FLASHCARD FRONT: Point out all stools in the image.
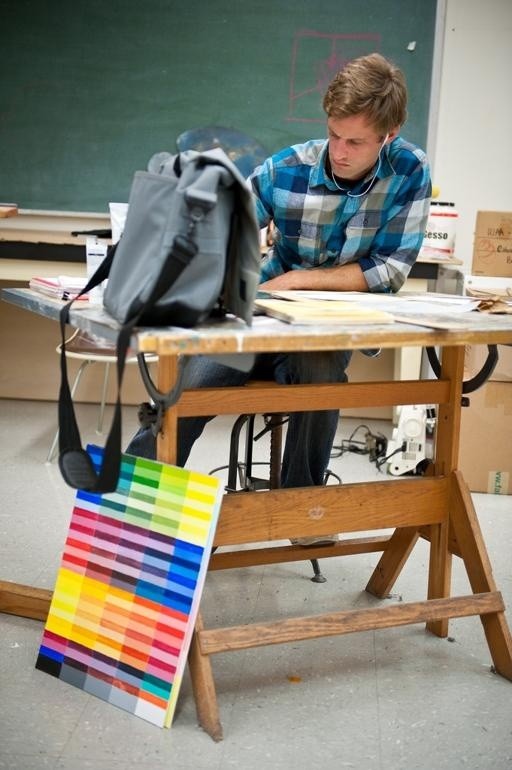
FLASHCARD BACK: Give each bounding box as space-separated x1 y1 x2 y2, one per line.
211 373 348 584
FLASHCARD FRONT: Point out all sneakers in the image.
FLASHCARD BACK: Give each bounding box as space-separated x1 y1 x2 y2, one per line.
289 532 338 547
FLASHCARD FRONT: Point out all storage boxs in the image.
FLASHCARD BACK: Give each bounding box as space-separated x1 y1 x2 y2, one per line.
471 209 511 277
434 344 512 495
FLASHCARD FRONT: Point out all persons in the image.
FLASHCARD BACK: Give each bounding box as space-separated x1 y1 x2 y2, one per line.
123 54 433 549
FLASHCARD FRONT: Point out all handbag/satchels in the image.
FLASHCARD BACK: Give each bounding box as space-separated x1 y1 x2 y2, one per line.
56 151 262 498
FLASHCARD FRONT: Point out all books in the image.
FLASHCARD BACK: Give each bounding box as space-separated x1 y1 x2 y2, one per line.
256 289 399 302
251 300 397 326
30 275 91 301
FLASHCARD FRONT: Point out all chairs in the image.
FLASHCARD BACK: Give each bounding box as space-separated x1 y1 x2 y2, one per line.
44 327 159 467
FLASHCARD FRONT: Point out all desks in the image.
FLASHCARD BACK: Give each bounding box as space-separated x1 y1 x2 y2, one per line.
0 287 512 743
